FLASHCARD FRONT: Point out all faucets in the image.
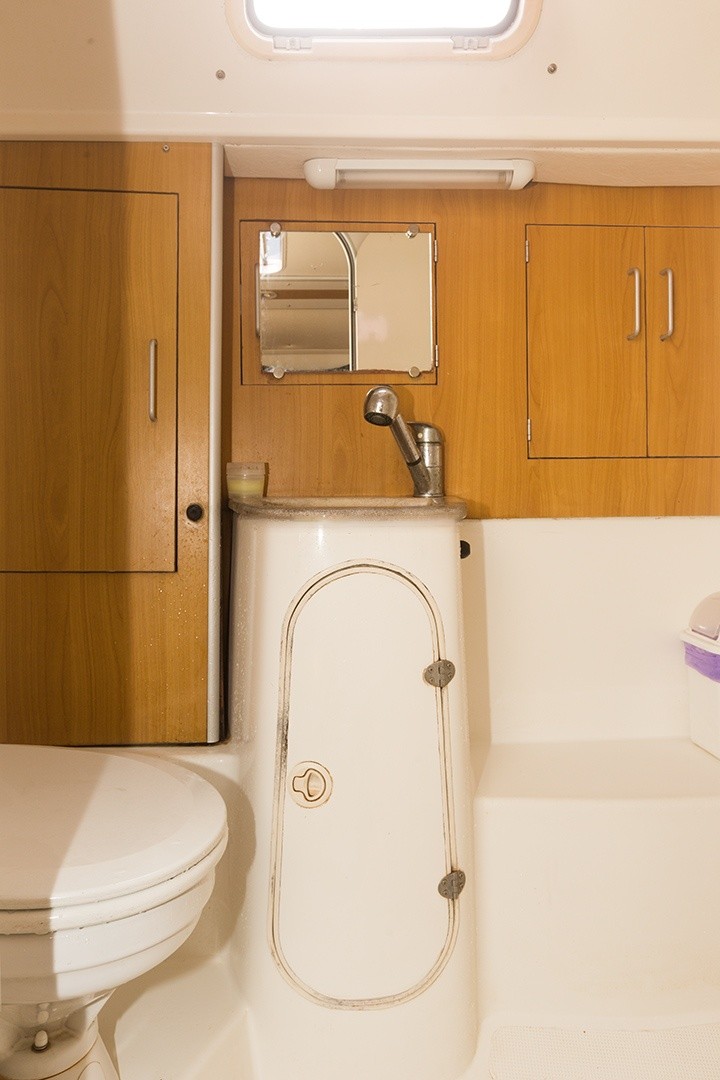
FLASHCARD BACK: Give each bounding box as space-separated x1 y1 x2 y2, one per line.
363 385 445 498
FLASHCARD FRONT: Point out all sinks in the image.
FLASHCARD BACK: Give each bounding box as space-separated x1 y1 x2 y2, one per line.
231 495 469 523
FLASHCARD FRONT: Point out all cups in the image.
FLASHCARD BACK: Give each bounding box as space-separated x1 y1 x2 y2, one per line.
225 461 265 500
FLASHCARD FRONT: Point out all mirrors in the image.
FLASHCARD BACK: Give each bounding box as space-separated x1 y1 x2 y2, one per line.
255 224 435 379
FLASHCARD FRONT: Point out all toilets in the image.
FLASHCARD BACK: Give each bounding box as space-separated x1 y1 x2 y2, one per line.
0 740 230 1080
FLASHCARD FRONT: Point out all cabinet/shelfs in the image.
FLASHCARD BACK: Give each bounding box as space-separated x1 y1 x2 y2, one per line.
0 138 226 746
223 177 720 517
